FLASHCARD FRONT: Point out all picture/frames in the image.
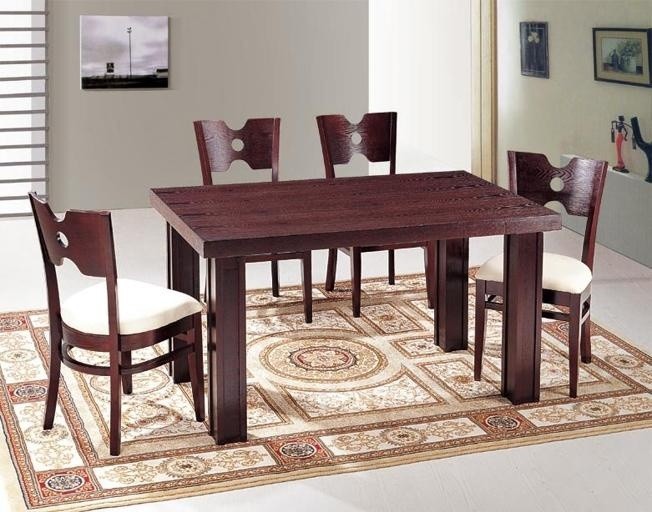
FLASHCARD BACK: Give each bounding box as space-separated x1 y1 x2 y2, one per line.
518 21 549 80
591 27 651 87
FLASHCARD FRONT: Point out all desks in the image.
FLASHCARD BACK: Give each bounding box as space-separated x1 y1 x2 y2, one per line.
148 169 564 446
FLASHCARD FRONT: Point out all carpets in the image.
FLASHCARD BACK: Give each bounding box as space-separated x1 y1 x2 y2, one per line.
0 266 652 510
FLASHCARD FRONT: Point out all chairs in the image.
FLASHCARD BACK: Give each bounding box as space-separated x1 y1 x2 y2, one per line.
193 118 312 322
316 113 436 317
27 191 204 456
470 149 608 400
